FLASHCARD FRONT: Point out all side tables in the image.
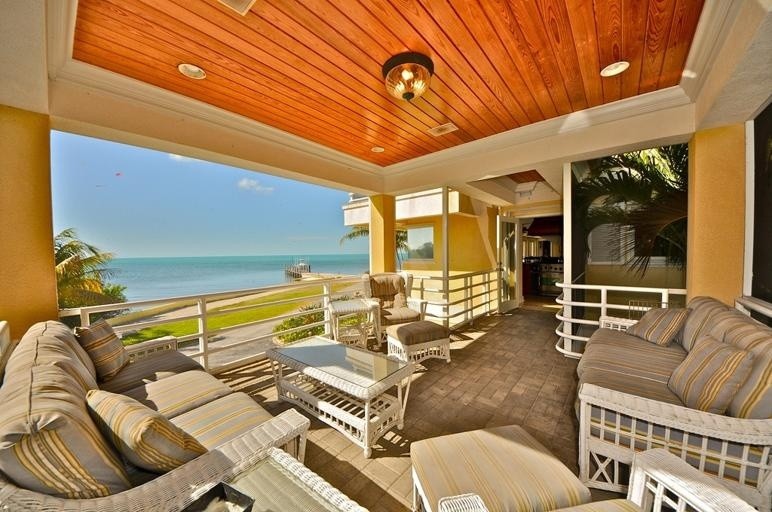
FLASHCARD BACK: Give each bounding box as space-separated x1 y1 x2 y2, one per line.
329 299 375 342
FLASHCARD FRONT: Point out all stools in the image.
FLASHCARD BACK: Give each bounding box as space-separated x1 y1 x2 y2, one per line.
387 320 452 366
412 426 592 510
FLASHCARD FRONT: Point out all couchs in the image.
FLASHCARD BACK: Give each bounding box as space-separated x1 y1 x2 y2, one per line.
574 296 770 494
0 319 310 512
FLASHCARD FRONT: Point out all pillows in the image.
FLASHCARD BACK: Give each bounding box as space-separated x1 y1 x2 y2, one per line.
85 389 209 475
625 308 691 348
667 335 754 415
74 317 131 382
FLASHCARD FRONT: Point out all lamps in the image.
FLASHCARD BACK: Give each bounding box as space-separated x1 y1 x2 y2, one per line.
383 53 434 103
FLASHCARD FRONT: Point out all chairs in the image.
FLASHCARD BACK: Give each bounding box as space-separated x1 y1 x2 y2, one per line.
363 272 428 348
